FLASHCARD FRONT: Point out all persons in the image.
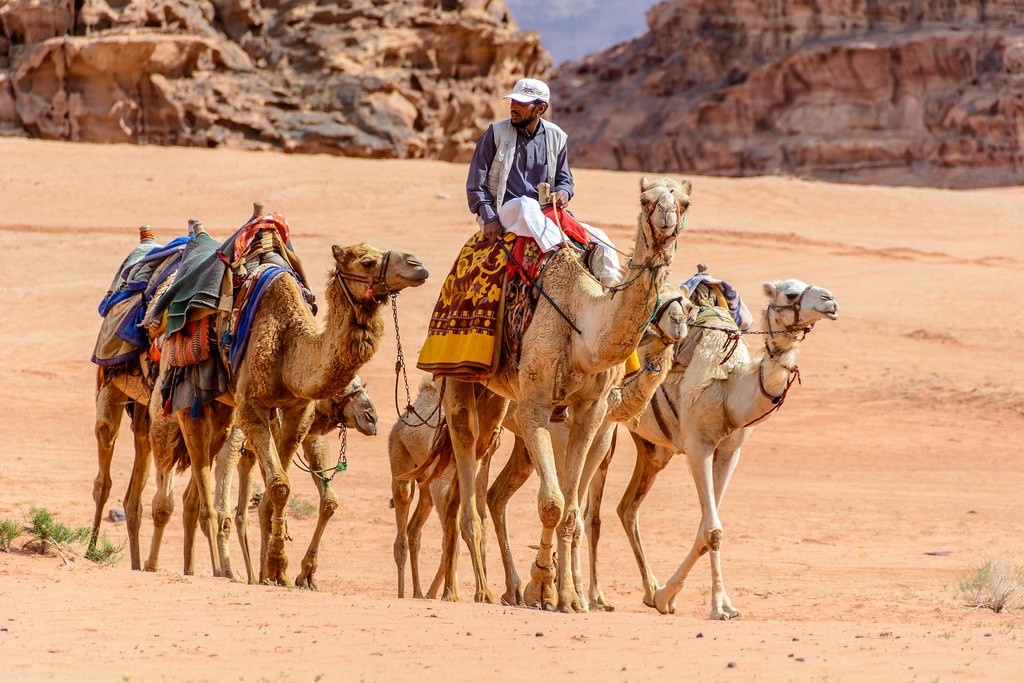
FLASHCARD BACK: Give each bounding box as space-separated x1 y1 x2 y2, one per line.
466 78 627 294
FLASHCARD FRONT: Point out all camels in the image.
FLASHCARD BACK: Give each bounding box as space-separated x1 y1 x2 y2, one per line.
81 202 429 594
388 176 840 620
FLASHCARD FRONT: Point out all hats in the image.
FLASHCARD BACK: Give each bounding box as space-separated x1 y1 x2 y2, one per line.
502 78 551 105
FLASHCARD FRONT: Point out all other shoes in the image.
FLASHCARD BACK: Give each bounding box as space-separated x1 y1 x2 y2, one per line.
583 241 604 280
